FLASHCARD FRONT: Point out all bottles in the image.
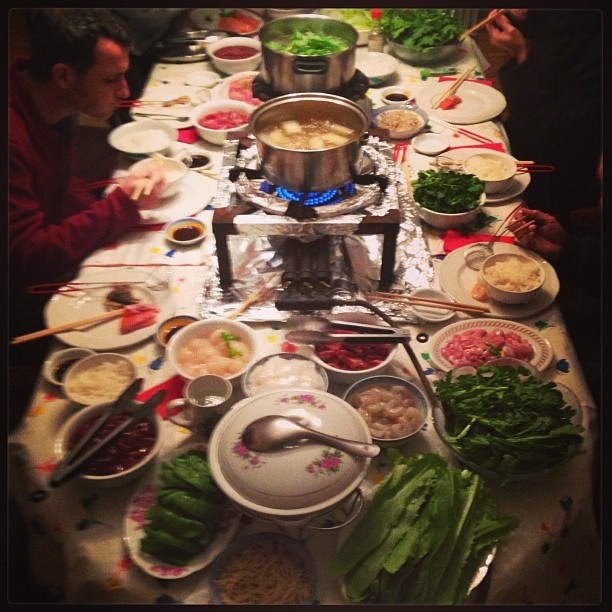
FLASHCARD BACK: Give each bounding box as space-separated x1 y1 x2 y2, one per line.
368 9 384 52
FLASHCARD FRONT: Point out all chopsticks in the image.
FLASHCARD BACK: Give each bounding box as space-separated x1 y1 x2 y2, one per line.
228 272 275 320
130 172 157 201
431 66 476 110
10 309 123 345
369 291 491 316
459 8 508 39
429 160 534 165
504 214 535 234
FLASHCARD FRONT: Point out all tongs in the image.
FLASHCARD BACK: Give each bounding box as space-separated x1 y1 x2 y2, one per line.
48 377 166 489
286 315 411 345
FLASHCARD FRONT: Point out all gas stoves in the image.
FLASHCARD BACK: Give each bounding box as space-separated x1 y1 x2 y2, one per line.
252 69 369 103
237 142 389 217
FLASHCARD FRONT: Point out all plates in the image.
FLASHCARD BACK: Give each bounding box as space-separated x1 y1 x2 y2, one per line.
42 347 96 385
220 9 265 35
371 104 429 140
180 151 213 171
380 86 414 104
43 277 163 351
211 71 264 110
330 480 497 605
267 8 300 18
156 17 208 63
184 69 220 88
103 167 215 224
208 532 318 605
439 242 561 318
129 85 211 129
428 318 553 382
107 120 179 156
156 315 199 349
411 132 449 155
415 80 507 124
436 148 531 203
164 217 208 246
280 14 329 19
409 288 457 322
123 443 244 580
432 358 583 482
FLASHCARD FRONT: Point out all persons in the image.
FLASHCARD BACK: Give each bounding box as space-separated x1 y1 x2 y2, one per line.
506 152 604 358
485 7 603 209
7 8 167 384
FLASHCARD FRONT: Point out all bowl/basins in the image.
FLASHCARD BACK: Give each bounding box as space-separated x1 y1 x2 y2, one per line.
309 312 398 385
242 352 329 399
413 191 486 228
465 153 517 195
481 252 546 305
207 37 262 76
127 157 187 199
343 374 428 448
207 388 372 530
355 28 377 46
54 401 166 489
191 99 253 146
386 38 459 65
62 353 137 407
357 51 399 87
165 317 259 386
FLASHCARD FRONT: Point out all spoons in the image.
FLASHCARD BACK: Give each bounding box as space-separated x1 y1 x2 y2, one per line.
129 112 188 122
242 415 381 458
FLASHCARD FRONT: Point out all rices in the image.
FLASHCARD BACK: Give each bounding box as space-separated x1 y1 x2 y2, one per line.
484 257 541 292
67 362 132 402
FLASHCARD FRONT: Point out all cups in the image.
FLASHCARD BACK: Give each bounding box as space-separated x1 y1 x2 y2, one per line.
165 375 233 432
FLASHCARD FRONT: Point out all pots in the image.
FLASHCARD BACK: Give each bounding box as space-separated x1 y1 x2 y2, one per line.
248 92 370 191
259 14 359 95
